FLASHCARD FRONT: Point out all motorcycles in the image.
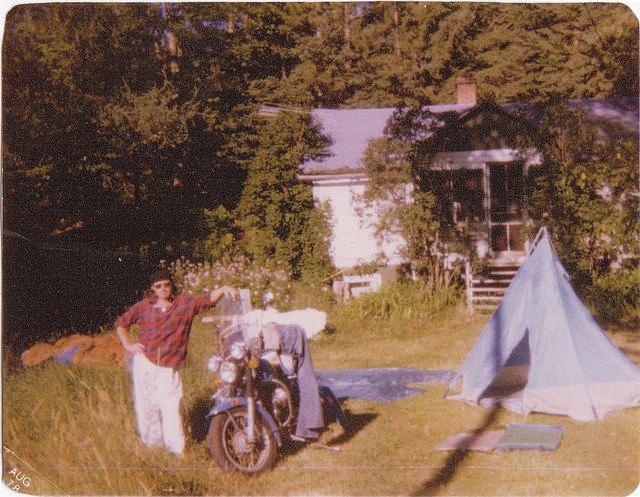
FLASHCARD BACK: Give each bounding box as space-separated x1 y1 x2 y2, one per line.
201 288 354 477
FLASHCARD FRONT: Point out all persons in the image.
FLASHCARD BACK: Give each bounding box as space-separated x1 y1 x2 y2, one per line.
112 265 240 465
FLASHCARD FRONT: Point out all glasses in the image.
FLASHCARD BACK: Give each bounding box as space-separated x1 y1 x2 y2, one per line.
152 283 171 289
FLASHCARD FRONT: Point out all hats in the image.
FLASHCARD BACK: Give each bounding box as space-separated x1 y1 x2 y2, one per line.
152 270 171 282
